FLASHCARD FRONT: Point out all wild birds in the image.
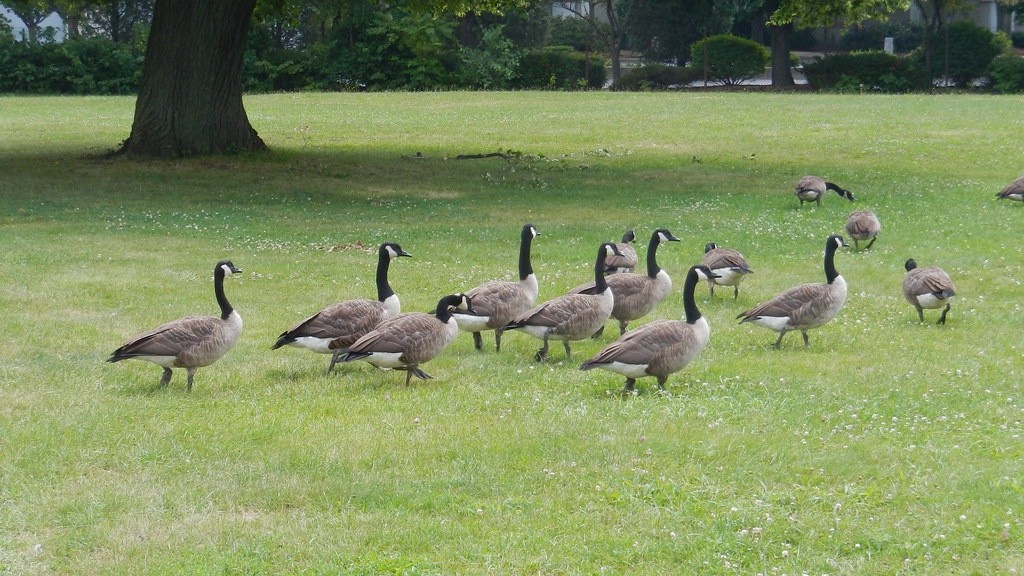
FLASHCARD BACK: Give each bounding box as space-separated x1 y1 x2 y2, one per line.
845 208 881 251
105 260 244 391
426 223 541 352
736 233 851 349
601 230 638 277
578 265 722 394
903 258 956 325
699 243 753 302
994 175 1024 204
334 293 478 388
496 242 625 363
792 175 857 208
565 229 681 339
269 242 412 373
